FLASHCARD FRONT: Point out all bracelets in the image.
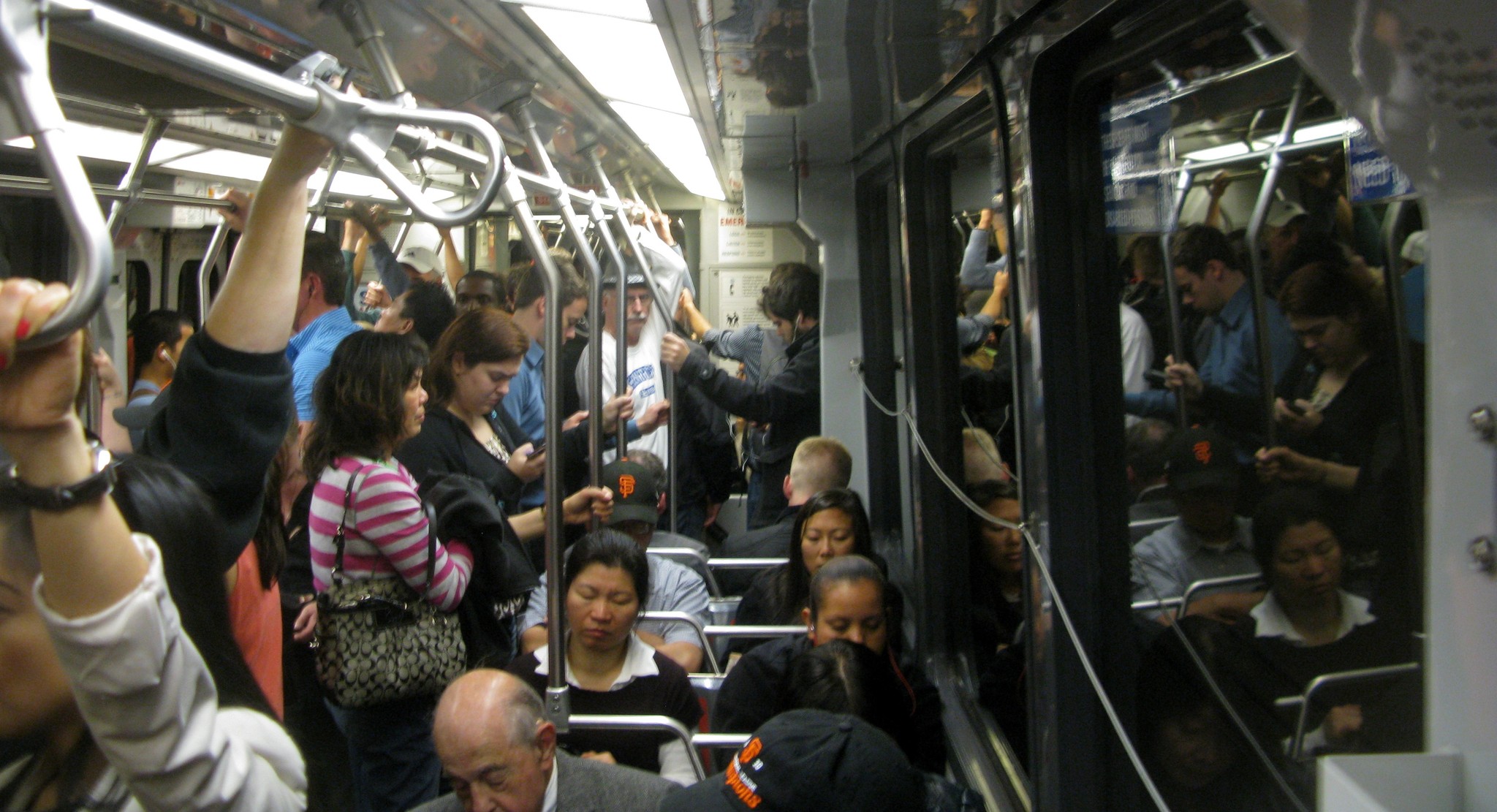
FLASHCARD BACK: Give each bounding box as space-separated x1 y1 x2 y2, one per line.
0 436 117 512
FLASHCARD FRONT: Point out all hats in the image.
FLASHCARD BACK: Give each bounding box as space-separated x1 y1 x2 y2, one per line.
601 257 646 284
1170 425 1241 491
599 457 659 523
660 709 915 812
624 451 668 490
394 247 443 276
1401 230 1426 264
1264 200 1308 226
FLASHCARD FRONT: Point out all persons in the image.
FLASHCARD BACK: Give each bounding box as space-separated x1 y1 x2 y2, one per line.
711 555 946 773
720 437 852 595
408 668 682 812
660 709 925 812
518 460 710 673
128 309 195 452
956 188 1151 753
504 529 704 773
660 261 820 537
719 489 904 672
211 189 693 812
0 277 310 812
74 73 365 716
1118 151 1429 812
784 639 985 812
628 449 710 588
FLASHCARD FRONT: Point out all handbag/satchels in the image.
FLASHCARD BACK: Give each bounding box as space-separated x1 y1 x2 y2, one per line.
308 467 465 711
